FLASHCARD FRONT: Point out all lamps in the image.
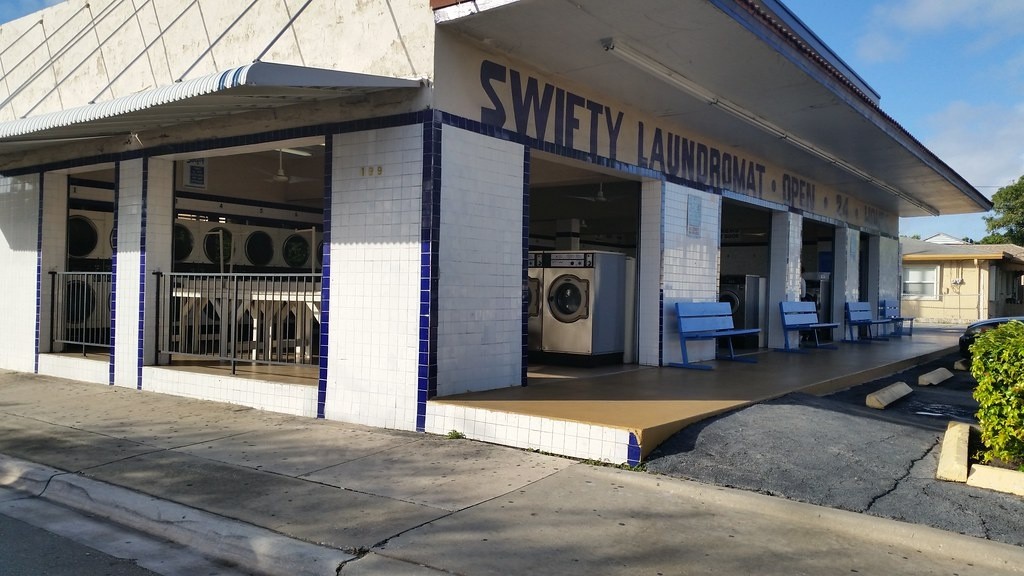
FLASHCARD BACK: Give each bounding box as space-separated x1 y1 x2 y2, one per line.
599 38 940 218
276 149 314 157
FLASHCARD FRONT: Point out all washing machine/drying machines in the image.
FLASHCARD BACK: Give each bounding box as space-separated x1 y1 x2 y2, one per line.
65 179 325 342
718 275 758 350
528 250 626 356
801 271 831 330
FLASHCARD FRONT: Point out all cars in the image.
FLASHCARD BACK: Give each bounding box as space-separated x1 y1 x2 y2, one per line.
958 315 1024 362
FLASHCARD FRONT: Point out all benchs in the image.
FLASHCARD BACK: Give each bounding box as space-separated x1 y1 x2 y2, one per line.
668 302 762 369
841 301 892 343
880 299 915 337
774 302 840 352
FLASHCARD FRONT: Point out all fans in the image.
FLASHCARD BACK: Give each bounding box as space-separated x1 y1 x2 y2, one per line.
250 148 324 183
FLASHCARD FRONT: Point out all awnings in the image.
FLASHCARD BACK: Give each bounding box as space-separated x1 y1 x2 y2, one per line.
0 62 426 154
